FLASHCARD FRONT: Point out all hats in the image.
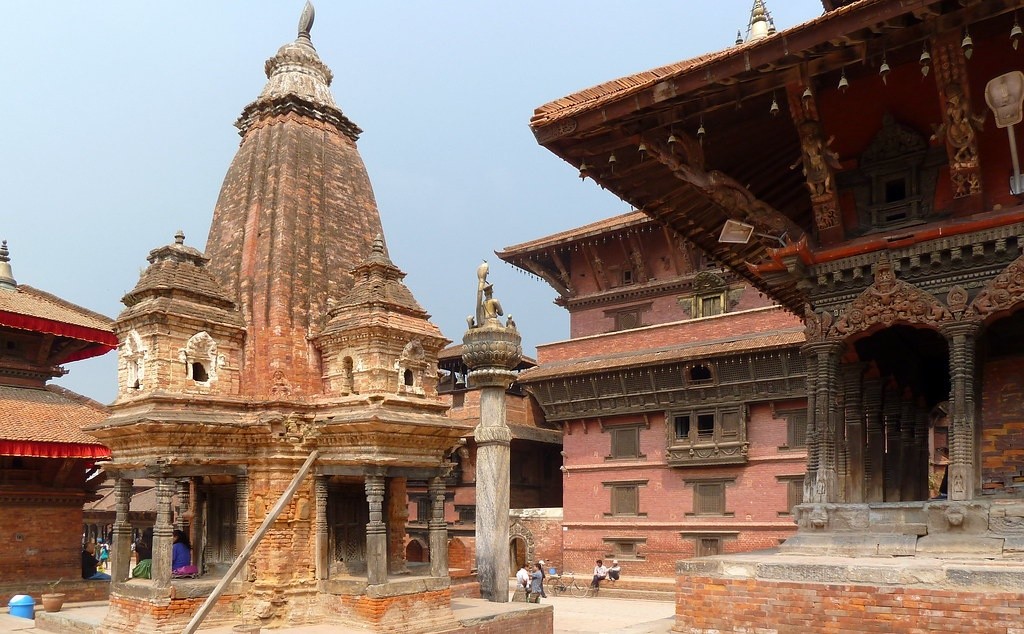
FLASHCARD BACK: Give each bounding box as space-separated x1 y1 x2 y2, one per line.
102 545 106 547
613 560 618 564
539 561 545 565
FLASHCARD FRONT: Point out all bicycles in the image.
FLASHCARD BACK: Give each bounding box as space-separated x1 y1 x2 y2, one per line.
547 571 588 598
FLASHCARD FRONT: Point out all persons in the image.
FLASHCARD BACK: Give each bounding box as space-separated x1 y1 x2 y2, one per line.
100 545 109 569
591 560 607 588
82 543 111 580
481 283 503 327
528 563 543 601
538 560 547 598
516 563 530 589
606 560 621 581
172 530 190 570
131 543 152 578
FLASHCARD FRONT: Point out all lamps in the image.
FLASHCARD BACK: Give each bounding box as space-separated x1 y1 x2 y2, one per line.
984 72 1024 193
718 220 779 246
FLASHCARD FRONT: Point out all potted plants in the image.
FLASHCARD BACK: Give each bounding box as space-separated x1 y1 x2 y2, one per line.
41 577 66 612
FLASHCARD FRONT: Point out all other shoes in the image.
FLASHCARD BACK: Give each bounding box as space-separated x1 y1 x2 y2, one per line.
612 578 615 582
542 595 547 598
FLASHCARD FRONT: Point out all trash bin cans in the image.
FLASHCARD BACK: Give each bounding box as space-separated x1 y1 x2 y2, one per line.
8 594 36 619
